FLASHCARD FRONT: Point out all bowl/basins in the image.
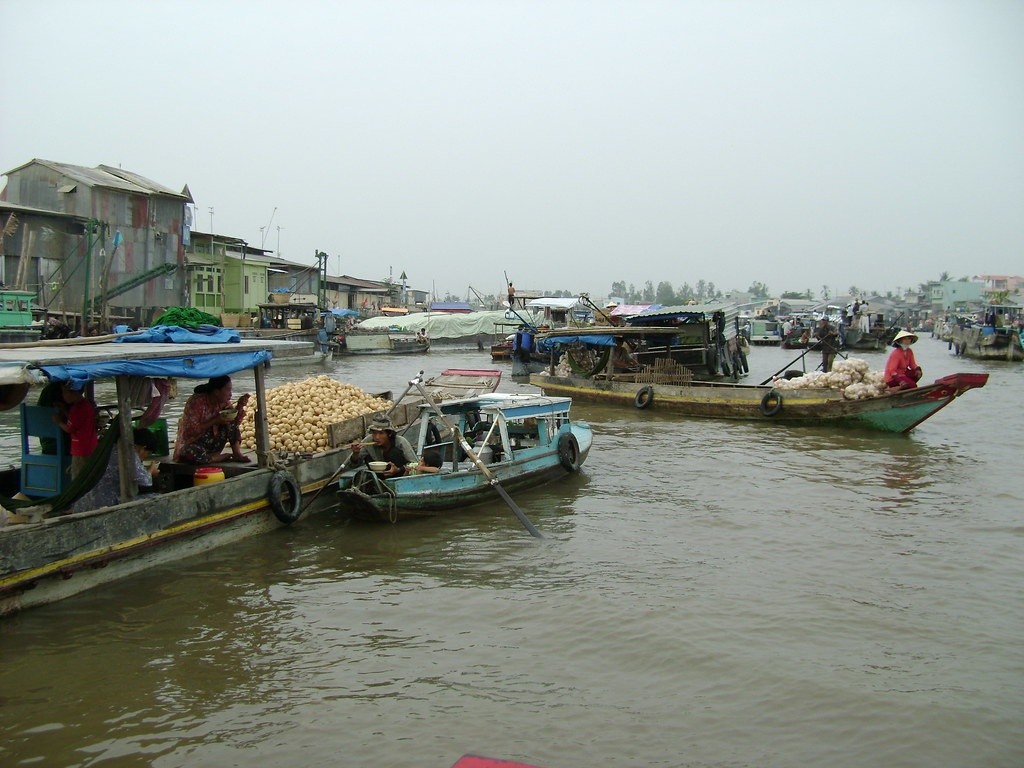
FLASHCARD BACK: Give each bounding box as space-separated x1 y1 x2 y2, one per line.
219 409 238 421
368 461 389 470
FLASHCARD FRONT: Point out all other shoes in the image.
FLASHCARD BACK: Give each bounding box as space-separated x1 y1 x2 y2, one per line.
913 367 921 378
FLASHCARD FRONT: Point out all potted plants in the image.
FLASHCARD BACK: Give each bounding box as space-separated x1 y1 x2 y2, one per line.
238 312 252 327
221 311 239 328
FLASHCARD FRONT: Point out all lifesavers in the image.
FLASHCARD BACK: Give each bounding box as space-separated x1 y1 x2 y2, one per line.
733 363 739 379
469 411 482 431
633 385 654 410
722 361 731 376
706 347 718 375
268 470 301 523
740 352 750 373
761 392 782 416
558 432 580 473
733 351 743 374
713 310 726 335
427 422 442 445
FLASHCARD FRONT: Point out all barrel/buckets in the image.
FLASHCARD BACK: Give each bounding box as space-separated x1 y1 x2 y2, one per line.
134 418 169 457
194 467 225 486
472 441 493 464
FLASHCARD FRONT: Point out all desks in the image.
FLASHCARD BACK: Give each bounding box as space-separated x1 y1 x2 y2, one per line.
256 302 314 330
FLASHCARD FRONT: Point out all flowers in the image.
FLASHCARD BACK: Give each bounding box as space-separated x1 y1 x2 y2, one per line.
272 288 291 294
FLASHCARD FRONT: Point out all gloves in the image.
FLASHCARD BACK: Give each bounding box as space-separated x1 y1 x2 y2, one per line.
900 381 910 390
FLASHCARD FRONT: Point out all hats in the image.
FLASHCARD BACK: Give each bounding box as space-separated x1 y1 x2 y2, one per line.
891 330 918 347
606 302 617 308
367 413 405 433
821 316 829 321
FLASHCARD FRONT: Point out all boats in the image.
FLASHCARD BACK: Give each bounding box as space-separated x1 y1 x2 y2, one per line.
0 342 502 617
491 296 1024 379
240 303 351 349
529 327 989 434
324 399 594 523
0 290 146 347
324 328 430 353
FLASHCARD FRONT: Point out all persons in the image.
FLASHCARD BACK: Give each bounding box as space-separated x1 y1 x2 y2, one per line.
813 316 840 372
362 297 429 317
735 297 880 351
174 376 251 466
260 307 361 358
887 303 1024 339
508 282 516 309
416 328 429 344
348 413 443 481
885 330 923 390
36 382 98 515
71 428 160 513
475 431 498 464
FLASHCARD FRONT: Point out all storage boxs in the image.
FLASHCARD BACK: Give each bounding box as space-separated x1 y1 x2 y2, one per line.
284 318 301 330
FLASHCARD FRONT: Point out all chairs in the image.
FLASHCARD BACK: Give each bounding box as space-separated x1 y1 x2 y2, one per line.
18 402 72 499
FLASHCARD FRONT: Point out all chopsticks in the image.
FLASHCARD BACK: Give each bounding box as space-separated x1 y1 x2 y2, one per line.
224 399 240 408
355 442 377 445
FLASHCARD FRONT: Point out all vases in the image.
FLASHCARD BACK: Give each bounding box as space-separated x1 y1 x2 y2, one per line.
272 293 290 304
